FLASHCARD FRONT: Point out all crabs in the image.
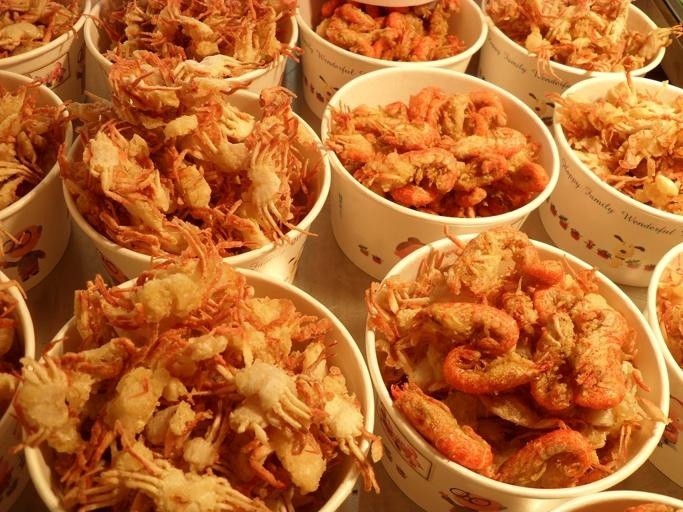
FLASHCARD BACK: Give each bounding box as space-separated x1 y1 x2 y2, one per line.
0 0 383 512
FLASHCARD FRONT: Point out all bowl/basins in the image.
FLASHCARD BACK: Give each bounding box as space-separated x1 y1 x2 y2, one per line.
552 490 682 511
297 2 491 120
59 84 332 288
363 230 670 512
316 63 564 282
471 1 668 136
1 266 38 510
1 70 74 294
2 1 83 102
642 243 683 489
20 265 378 510
537 74 683 285
84 0 299 108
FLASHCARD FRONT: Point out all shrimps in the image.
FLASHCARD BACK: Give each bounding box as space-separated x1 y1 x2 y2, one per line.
308 0 670 512
